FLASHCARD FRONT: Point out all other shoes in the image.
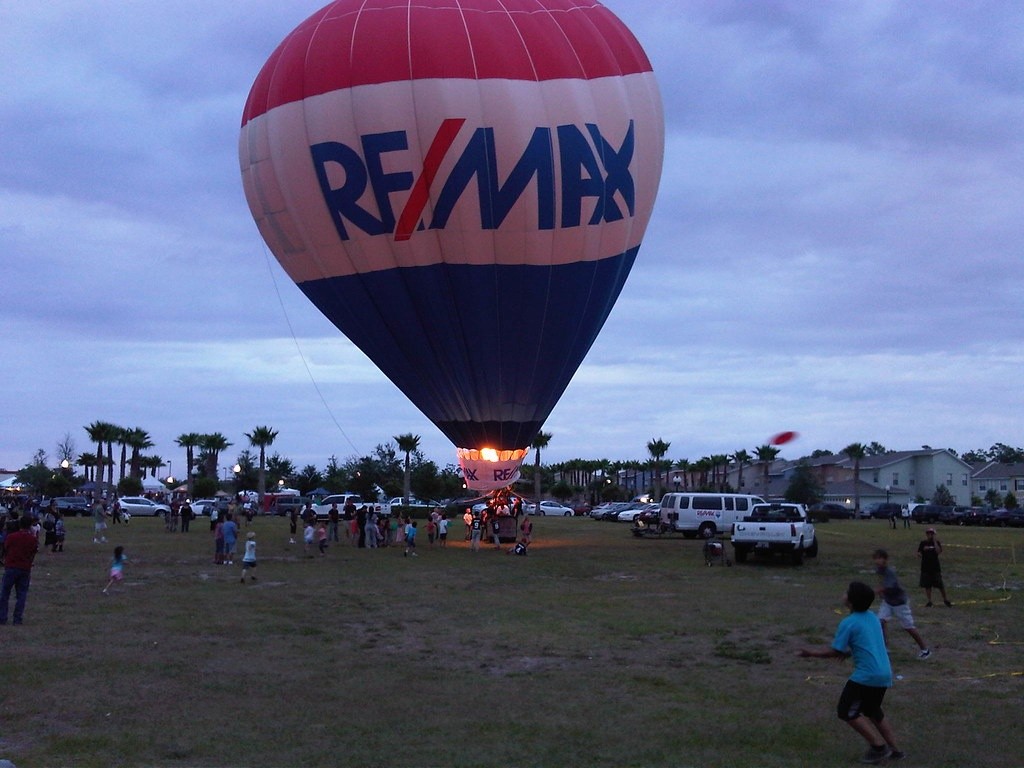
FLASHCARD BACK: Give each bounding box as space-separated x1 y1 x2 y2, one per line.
94 539 100 544
404 551 408 557
213 559 233 565
101 539 108 542
925 602 934 608
241 576 245 584
103 589 109 596
0 622 8 625
944 600 953 608
251 576 257 580
412 553 418 557
13 621 22 626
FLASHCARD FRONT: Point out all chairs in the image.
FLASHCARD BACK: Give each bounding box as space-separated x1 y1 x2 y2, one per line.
758 508 768 515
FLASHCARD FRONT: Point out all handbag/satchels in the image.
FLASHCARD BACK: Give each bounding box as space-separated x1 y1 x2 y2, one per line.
43 520 54 530
211 510 218 521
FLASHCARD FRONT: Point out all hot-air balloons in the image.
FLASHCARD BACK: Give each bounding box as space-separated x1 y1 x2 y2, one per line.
236 0 664 542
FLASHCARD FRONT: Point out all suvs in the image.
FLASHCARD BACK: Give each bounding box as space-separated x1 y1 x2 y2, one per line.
40 496 92 517
107 496 172 518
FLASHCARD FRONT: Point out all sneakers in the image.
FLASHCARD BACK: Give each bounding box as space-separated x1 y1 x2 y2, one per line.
860 747 892 763
917 648 932 660
890 750 906 761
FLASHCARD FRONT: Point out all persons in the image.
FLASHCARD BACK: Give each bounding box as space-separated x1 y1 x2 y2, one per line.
208 496 241 565
145 489 164 504
301 502 329 555
169 498 194 532
422 508 449 550
793 581 908 763
0 493 67 568
0 515 40 625
396 506 418 557
102 546 131 595
92 497 131 544
240 532 258 584
891 514 898 529
343 497 394 549
288 508 298 544
916 527 953 607
327 502 340 543
868 549 932 659
463 496 533 556
901 505 912 529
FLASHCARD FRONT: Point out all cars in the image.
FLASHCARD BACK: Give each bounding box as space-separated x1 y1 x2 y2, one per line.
178 499 218 520
391 494 659 527
810 503 1024 528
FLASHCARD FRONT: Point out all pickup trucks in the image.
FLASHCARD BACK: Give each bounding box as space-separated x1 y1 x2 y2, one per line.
300 495 391 519
731 503 818 567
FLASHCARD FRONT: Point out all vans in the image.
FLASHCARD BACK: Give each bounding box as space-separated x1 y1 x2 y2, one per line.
269 496 315 518
659 492 768 540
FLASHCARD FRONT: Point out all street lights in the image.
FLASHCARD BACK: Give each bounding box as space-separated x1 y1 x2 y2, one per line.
884 485 891 502
222 466 227 483
167 460 172 478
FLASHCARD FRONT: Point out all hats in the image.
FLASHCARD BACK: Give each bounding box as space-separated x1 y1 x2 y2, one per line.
926 528 936 534
522 538 526 543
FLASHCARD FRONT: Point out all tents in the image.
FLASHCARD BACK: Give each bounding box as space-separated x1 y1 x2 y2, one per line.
142 475 166 498
